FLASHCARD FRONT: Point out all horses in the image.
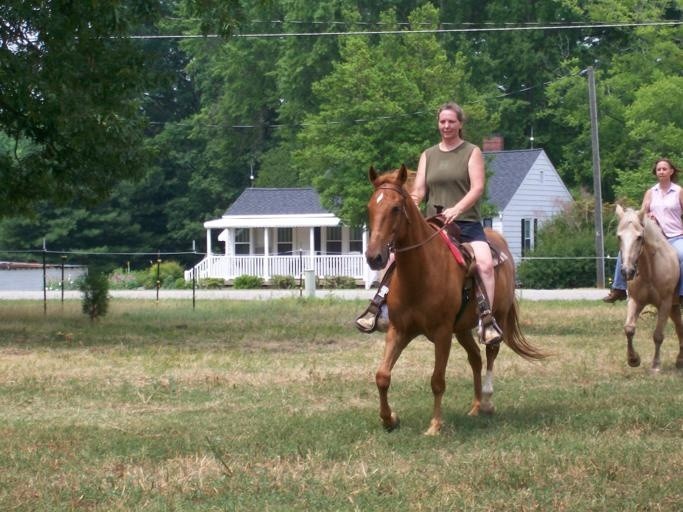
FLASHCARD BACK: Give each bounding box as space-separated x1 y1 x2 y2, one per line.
365 162 550 436
615 203 683 375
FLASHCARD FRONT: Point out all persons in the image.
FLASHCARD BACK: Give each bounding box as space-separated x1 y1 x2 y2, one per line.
603 157 682 305
355 102 504 344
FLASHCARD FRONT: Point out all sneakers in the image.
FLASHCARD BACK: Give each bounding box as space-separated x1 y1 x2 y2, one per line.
478 318 504 346
603 288 626 304
356 311 388 333
